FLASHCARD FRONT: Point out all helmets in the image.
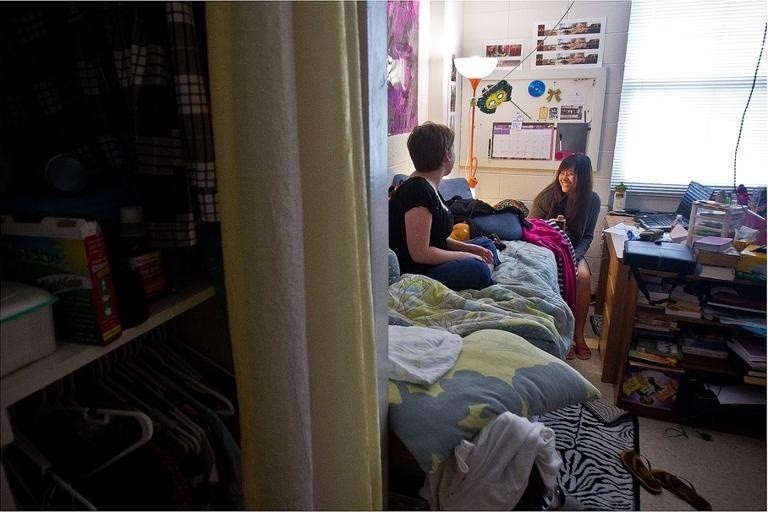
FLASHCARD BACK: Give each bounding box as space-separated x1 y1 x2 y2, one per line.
493 199 529 218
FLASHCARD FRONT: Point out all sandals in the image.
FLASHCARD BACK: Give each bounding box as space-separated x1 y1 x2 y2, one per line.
567 336 591 360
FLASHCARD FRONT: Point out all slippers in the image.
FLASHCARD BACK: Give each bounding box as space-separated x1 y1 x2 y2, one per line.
653 469 711 510
621 449 661 494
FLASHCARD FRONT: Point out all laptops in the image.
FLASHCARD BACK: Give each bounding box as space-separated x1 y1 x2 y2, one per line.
636 180 715 231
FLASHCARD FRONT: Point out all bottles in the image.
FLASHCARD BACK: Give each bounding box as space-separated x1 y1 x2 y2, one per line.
671 215 685 233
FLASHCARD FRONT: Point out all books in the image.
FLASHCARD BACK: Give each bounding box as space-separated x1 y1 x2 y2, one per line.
628 236 767 386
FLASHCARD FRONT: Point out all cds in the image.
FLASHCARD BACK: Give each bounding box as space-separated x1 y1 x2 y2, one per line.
528 80 545 97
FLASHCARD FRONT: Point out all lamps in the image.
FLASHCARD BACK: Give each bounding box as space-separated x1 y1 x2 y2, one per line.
452 55 499 186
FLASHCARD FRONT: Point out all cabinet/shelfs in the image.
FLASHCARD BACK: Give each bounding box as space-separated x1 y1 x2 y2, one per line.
588 214 768 419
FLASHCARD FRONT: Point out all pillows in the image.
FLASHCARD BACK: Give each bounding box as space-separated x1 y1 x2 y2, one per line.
396 174 523 241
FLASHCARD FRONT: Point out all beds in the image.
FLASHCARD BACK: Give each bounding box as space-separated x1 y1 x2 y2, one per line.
383 214 569 511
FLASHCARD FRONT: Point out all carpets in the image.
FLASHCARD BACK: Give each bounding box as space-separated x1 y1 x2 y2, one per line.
525 400 641 511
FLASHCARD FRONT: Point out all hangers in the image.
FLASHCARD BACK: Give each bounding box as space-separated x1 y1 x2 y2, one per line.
9 305 240 511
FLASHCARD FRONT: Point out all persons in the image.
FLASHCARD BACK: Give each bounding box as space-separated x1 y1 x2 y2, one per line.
389 121 499 291
526 153 600 359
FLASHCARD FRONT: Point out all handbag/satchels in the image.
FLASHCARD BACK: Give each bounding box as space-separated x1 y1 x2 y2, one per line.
623 240 695 274
